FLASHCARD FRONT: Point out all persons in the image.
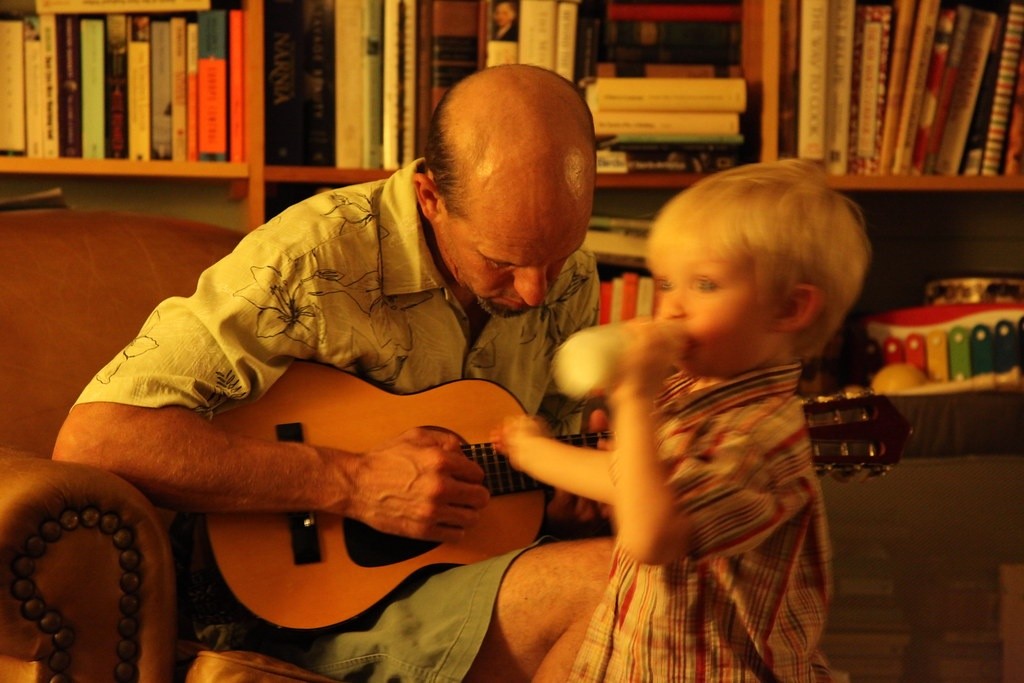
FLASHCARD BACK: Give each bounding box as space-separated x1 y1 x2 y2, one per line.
492 160 872 683
51 64 612 683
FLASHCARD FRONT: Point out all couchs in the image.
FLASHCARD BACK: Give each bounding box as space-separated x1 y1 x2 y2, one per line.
0 210 329 683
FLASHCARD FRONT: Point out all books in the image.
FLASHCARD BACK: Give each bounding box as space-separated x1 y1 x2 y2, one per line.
0 0 1024 323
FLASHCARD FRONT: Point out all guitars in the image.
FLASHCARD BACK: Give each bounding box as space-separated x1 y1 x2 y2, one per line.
203 356 915 637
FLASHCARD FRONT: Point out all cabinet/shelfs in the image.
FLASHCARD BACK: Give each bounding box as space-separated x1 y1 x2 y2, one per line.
0 0 1024 234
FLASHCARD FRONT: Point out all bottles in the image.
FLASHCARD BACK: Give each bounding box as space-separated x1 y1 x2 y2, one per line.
552 317 688 401
